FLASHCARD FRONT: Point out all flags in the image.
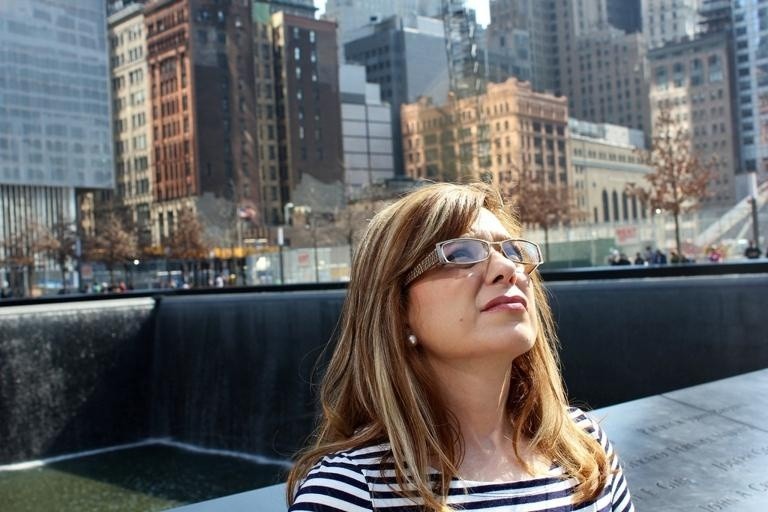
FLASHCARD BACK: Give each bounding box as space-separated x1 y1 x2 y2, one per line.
237 204 256 219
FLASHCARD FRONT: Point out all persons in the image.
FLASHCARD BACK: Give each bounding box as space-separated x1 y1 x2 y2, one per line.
58 284 71 294
285 180 637 512
608 246 696 266
0 280 20 298
745 239 762 259
81 271 228 293
708 246 722 262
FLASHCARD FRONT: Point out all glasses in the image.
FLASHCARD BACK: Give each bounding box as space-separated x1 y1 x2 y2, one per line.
402 238 544 286
